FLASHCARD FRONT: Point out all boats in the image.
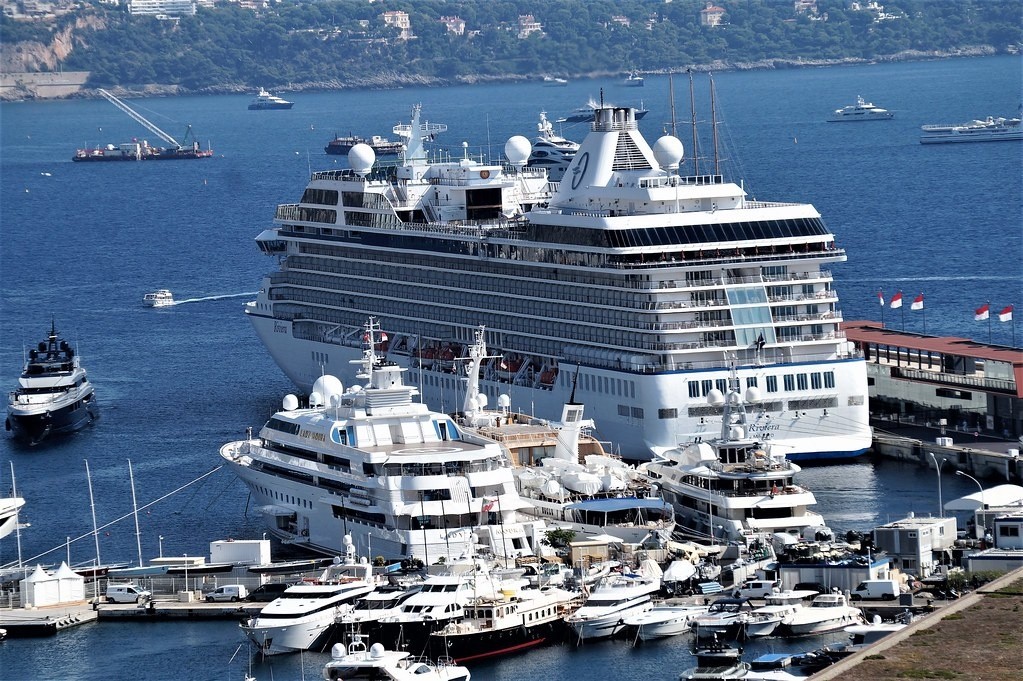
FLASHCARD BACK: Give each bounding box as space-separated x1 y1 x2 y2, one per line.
919 104 1023 144
543 76 567 88
142 289 174 308
324 131 402 156
540 366 560 387
216 314 547 572
6 311 99 445
566 99 650 123
494 359 520 380
0 351 1021 680
72 87 213 161
434 347 455 371
414 348 432 368
625 65 644 86
247 87 295 110
825 95 893 122
363 332 388 351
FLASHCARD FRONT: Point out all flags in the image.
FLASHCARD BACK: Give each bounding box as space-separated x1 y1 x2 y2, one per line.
482 499 494 514
451 362 456 373
382 333 388 342
501 359 507 370
878 291 884 306
889 291 902 309
755 334 765 352
363 332 369 342
998 305 1013 323
975 304 989 321
910 294 924 310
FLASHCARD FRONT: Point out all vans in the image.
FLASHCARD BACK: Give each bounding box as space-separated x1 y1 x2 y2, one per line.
732 580 781 599
794 581 833 601
850 580 901 601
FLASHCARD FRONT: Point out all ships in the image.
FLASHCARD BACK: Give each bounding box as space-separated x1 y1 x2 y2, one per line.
243 98 874 466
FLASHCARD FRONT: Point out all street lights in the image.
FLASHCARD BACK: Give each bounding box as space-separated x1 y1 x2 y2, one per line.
708 456 721 546
956 470 985 533
929 452 946 518
